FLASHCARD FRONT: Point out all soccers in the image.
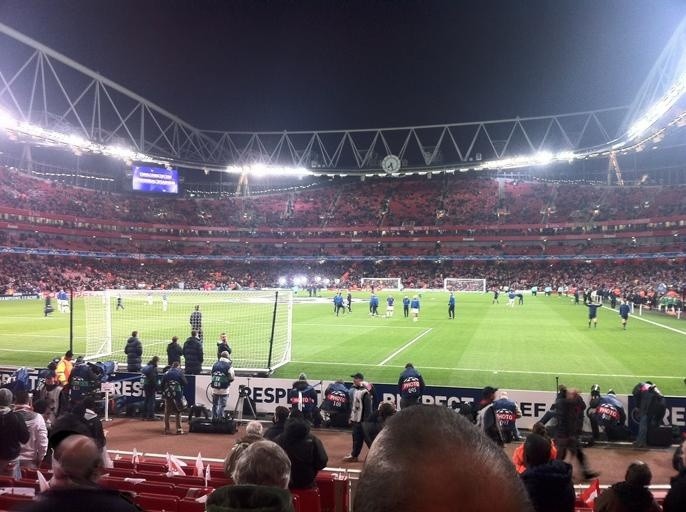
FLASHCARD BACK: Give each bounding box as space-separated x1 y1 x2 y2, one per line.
570 299 574 302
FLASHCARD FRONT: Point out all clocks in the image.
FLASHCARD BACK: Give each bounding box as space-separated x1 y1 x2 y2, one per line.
380 154 401 174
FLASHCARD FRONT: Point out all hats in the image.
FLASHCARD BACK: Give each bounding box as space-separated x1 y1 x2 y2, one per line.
500 391 508 400
482 386 498 398
351 373 364 380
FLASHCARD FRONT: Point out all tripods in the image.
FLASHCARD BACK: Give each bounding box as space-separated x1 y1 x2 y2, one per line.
234 398 257 418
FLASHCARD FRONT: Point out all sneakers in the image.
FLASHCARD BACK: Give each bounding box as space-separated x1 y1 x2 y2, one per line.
342 454 358 462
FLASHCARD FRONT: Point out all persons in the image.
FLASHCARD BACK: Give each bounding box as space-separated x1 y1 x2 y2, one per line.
511 372 686 512
358 172 686 331
0 330 358 512
1 159 357 319
190 305 203 343
357 363 511 462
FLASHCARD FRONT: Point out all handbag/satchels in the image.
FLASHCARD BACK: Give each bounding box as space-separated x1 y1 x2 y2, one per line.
188 417 237 435
173 395 188 412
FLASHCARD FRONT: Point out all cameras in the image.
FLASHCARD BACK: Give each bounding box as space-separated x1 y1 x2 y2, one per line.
238 385 247 398
590 384 601 397
